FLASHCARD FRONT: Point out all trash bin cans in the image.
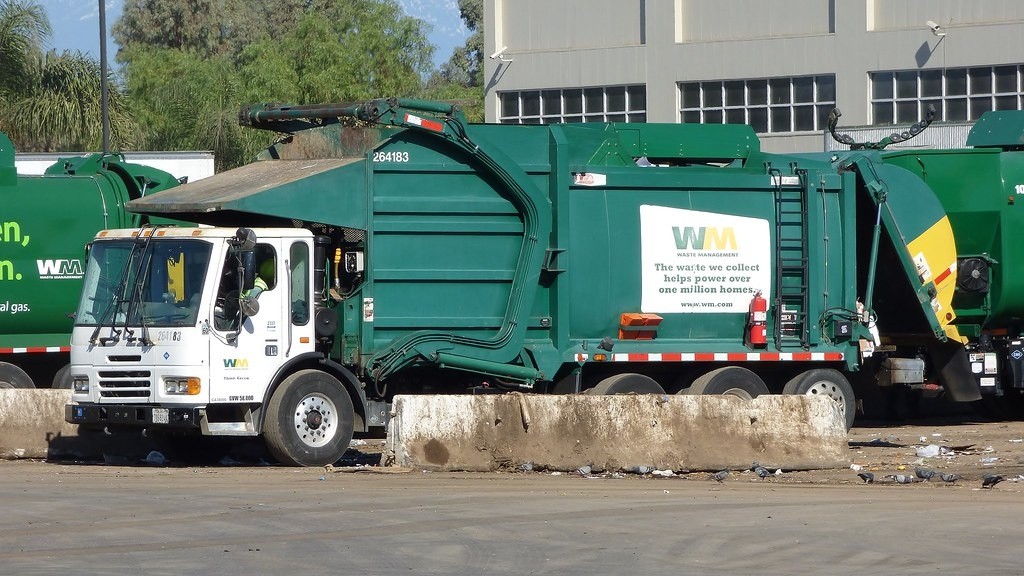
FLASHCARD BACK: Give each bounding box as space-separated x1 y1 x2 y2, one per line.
989 334 1024 390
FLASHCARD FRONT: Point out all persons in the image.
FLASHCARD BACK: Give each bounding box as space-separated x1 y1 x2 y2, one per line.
241 262 268 299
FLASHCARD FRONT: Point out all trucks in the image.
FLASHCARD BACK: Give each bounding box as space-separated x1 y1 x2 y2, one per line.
0 132 200 389
63 96 981 467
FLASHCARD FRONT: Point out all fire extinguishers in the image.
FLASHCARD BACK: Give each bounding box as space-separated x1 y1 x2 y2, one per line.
742 292 766 346
